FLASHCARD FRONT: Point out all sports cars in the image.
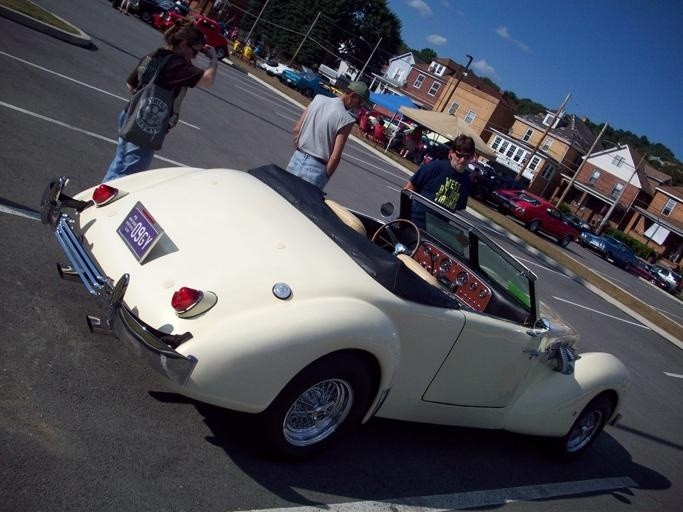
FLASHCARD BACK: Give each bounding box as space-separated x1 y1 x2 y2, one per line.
35 164 635 462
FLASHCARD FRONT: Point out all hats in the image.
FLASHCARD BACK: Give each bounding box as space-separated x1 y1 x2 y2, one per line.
347 81 373 109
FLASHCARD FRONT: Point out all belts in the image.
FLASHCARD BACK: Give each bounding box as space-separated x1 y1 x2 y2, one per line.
295 146 328 166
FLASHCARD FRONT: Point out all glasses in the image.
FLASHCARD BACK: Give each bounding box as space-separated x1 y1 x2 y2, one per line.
452 148 473 160
187 44 199 57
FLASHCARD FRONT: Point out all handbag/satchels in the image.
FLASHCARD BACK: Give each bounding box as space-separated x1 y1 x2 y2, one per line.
119 83 174 150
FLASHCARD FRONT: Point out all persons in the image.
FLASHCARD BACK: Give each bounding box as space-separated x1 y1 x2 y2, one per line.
98 19 217 186
119 0 132 17
355 110 369 138
405 134 475 248
249 45 256 66
111 1 121 11
374 120 387 146
284 81 374 192
399 125 422 159
390 127 405 148
